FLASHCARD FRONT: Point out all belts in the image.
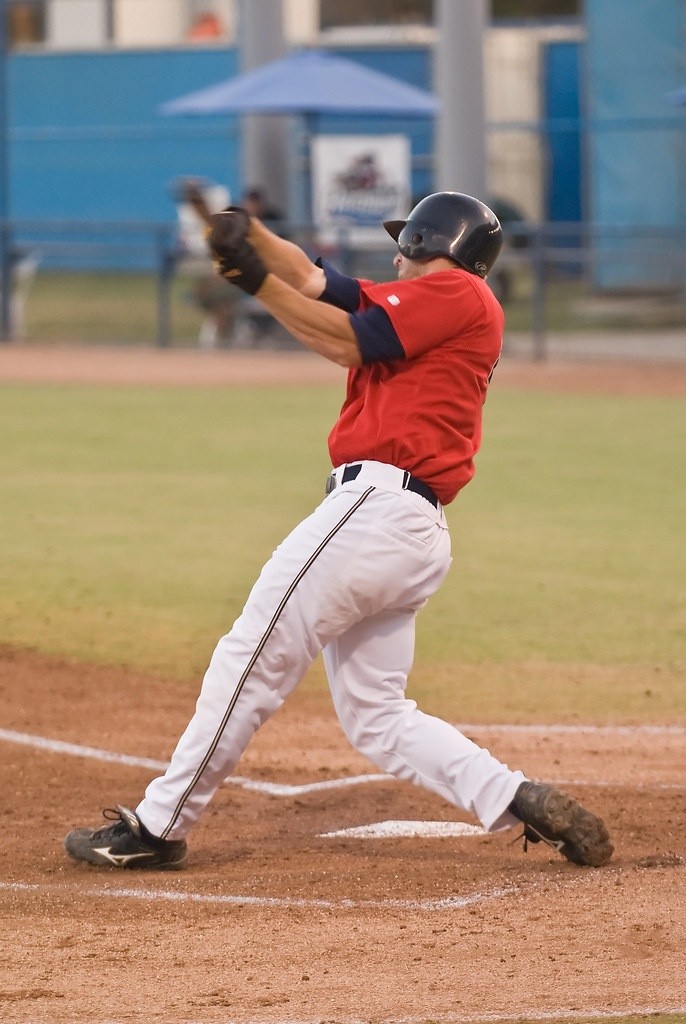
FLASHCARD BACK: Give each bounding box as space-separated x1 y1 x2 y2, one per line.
327 465 442 506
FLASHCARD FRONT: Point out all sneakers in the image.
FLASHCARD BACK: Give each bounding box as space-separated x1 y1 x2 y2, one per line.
508 782 614 867
66 805 188 872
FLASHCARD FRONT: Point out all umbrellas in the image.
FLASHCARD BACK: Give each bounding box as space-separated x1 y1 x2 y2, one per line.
162 48 441 262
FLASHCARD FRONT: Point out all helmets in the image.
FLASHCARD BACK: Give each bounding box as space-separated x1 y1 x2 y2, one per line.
384 191 505 277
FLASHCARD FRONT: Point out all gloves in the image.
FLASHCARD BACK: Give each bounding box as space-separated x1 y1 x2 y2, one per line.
207 205 268 297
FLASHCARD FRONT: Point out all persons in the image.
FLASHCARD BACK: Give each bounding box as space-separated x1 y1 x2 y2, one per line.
170 173 295 355
64 192 614 872
